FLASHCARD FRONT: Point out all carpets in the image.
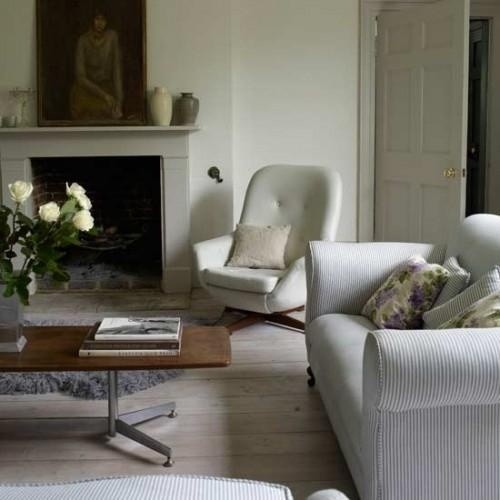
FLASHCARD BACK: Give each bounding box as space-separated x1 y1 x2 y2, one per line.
0 324 188 402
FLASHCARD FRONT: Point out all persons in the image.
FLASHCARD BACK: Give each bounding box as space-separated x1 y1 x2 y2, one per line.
67 0 124 120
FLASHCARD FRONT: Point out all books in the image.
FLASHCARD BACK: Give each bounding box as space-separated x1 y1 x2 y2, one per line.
78 317 183 358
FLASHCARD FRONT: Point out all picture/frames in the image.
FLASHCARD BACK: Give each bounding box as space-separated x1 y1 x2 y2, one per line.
34 0 150 127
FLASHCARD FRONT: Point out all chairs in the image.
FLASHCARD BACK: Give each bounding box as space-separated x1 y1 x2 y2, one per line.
191 160 343 333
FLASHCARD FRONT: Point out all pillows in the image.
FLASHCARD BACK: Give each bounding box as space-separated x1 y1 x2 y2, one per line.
225 221 293 273
363 251 500 338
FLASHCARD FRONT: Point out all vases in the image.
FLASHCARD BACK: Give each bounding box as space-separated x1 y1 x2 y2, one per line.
172 92 200 126
0 284 28 353
151 85 174 126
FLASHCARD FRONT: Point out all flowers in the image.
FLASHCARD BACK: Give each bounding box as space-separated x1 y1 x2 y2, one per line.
0 172 134 300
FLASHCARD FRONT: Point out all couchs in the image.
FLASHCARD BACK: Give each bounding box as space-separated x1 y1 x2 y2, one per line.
303 206 500 500
2 476 349 499
